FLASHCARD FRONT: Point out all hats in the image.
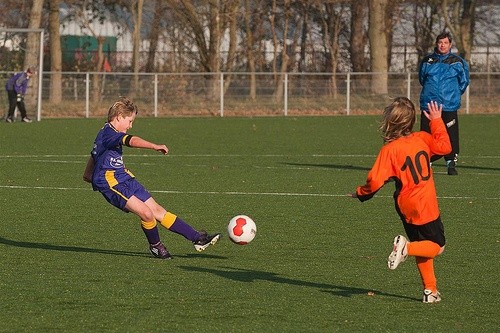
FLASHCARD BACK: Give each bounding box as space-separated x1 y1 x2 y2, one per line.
28 66 35 74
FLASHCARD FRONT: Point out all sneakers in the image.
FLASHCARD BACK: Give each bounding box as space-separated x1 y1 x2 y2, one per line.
149 241 172 259
193 230 221 253
388 235 410 270
422 289 443 304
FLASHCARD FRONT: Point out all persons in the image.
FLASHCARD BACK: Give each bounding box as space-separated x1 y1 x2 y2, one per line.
83 97 223 259
419 32 469 175
6 67 35 123
347 97 452 303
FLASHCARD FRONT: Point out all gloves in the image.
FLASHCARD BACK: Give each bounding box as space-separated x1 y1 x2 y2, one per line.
16 94 22 102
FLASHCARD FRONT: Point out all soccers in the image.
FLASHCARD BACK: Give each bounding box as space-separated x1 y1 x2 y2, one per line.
227 214 257 244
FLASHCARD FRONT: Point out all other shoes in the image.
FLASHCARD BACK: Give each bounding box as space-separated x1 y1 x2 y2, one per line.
6 116 14 123
22 116 34 123
446 160 458 175
